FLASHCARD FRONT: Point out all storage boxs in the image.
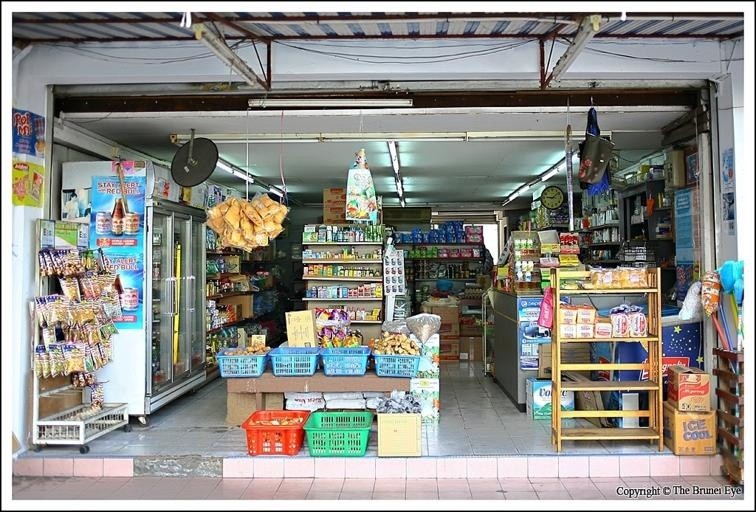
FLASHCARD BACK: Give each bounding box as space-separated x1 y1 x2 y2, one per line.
666 364 714 414
321 185 348 223
413 379 440 422
523 374 581 423
662 399 720 459
377 412 421 458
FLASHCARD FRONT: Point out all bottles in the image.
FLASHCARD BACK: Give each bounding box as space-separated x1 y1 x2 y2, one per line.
514 239 534 282
346 306 359 320
110 197 124 236
113 272 123 310
344 265 380 277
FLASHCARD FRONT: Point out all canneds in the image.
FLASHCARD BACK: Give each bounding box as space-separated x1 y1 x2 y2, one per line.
124 287 138 311
124 213 139 235
95 212 112 234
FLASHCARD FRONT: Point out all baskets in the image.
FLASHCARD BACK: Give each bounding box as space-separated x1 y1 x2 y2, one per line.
371 347 421 379
303 410 375 458
268 346 323 377
215 346 272 379
241 410 312 456
320 346 371 377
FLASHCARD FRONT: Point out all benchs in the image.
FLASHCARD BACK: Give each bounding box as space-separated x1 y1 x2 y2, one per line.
227 374 412 412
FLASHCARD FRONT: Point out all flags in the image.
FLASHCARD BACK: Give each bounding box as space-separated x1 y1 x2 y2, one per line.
577 132 617 185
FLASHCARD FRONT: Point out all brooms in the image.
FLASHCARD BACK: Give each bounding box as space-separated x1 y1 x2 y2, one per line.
718 260 744 458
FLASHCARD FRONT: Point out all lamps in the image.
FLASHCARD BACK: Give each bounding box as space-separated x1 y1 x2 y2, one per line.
165 125 619 149
248 94 418 115
501 141 584 204
386 137 410 209
542 10 610 85
189 18 261 85
214 154 289 199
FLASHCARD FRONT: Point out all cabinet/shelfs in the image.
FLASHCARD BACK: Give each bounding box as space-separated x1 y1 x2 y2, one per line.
204 206 299 367
497 208 587 291
24 220 138 456
300 219 498 376
570 157 697 307
547 262 671 456
713 342 744 495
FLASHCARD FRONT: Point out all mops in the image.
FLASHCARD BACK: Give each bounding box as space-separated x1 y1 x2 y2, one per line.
679 271 737 373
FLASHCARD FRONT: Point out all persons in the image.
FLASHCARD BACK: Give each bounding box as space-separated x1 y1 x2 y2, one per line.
62 193 81 219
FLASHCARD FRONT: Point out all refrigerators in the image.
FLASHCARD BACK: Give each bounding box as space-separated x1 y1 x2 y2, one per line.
59 158 208 426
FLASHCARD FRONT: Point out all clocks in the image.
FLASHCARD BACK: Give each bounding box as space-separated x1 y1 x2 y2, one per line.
539 182 568 211
